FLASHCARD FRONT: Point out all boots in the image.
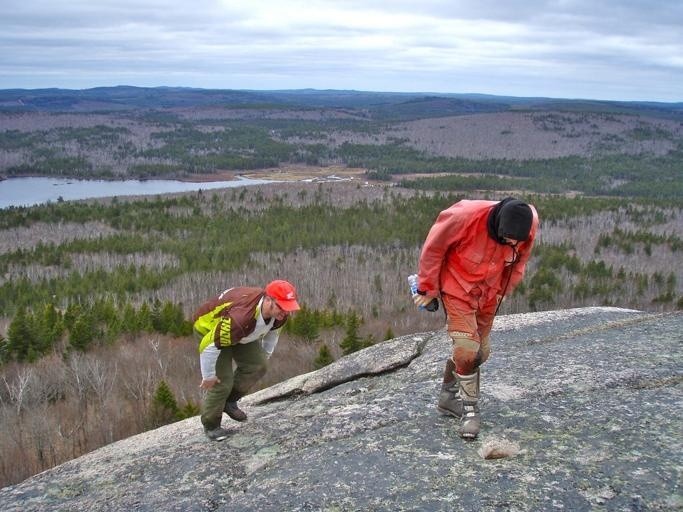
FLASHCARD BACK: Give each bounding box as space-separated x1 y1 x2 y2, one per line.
437 358 482 438
203 420 229 440
222 391 247 422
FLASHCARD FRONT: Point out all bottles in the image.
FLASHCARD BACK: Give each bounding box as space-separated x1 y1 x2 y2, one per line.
407 273 424 311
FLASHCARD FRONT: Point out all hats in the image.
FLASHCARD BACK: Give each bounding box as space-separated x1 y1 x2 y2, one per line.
265 280 301 312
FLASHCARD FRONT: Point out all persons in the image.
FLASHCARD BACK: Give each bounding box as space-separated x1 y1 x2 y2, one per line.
193 280 300 441
412 196 539 437
